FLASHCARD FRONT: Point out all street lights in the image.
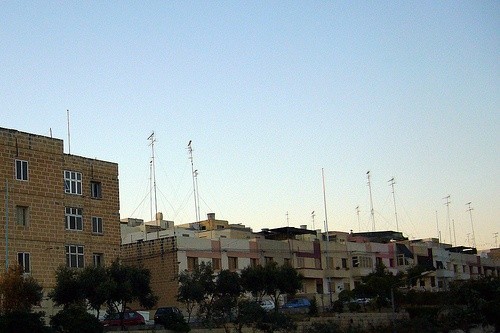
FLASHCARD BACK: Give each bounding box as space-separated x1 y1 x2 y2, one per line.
390 271 433 312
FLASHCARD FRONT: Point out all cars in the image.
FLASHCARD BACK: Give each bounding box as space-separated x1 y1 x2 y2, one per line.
154 307 184 325
256 300 275 312
101 311 145 326
282 298 311 309
